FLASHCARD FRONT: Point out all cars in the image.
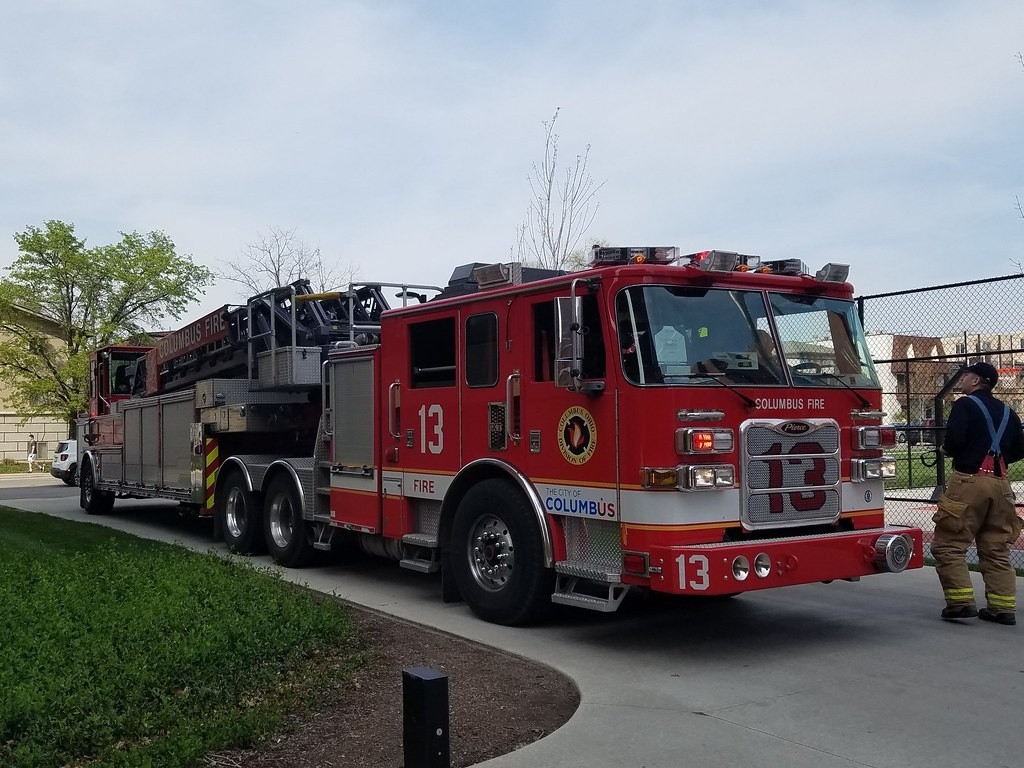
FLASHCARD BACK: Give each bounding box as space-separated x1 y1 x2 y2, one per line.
888 421 907 443
49 438 78 488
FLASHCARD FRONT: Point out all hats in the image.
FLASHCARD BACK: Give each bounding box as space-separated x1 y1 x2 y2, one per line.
958 362 999 386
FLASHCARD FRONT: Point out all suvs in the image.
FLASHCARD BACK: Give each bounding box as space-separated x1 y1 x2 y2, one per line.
905 418 950 446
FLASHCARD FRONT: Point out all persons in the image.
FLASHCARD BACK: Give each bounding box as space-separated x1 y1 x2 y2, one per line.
743 330 798 384
27 434 45 474
930 362 1024 625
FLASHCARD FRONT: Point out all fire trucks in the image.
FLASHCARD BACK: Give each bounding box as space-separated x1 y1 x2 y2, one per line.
71 242 928 628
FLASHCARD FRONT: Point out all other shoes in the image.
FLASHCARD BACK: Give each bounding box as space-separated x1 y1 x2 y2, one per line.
940 605 978 623
978 608 1017 625
